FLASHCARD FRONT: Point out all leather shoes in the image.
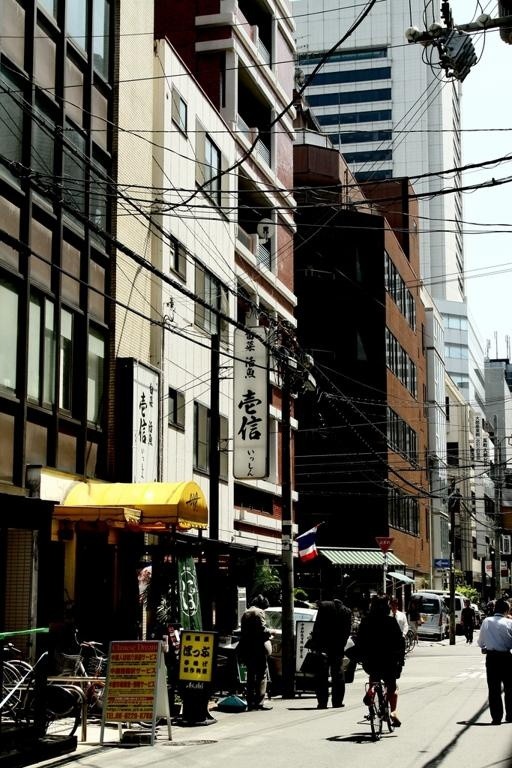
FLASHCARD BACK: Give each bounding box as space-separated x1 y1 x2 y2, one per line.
256 704 273 711
317 705 327 709
333 705 344 708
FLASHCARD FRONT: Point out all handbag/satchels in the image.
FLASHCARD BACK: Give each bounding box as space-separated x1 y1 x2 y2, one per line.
300 651 329 676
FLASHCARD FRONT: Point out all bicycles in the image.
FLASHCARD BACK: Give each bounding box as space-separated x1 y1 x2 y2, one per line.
404 629 420 655
0 624 168 730
364 672 395 741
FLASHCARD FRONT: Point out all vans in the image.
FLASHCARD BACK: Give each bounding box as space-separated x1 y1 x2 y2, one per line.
411 589 481 642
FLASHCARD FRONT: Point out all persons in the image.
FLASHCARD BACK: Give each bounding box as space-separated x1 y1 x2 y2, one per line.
405 599 422 644
477 598 511 726
387 598 409 673
353 594 406 727
310 583 351 709
232 593 275 711
460 600 476 645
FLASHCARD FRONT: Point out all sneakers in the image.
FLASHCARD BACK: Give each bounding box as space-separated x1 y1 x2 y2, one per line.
363 689 375 705
390 712 401 727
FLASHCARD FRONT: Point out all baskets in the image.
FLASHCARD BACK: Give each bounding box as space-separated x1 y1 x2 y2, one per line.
56 652 83 674
88 656 105 676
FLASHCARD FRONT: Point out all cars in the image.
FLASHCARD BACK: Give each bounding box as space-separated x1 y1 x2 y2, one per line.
233 607 321 698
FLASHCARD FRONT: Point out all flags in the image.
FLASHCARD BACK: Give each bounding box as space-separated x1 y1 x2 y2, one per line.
293 525 319 561
